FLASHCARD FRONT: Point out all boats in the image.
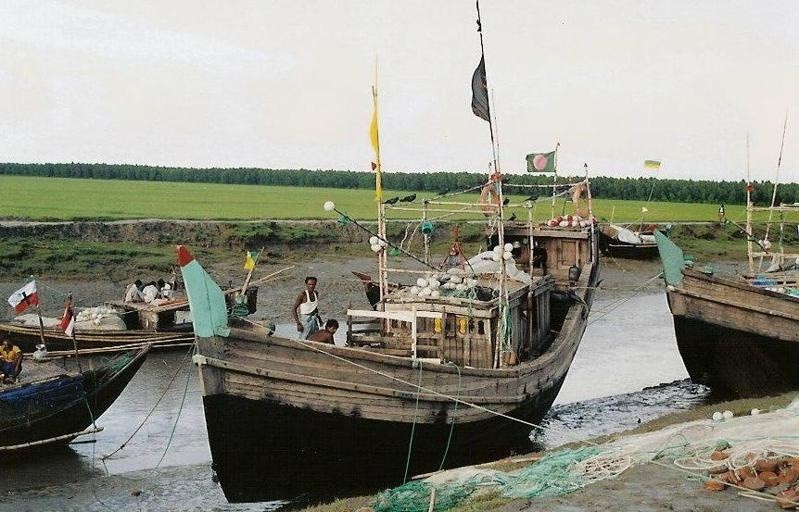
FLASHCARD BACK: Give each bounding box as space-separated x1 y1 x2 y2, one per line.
175 215 599 503
1 343 151 457
2 281 275 351
648 224 798 396
595 222 668 259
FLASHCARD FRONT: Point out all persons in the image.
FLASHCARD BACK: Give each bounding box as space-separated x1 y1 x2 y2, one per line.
719 204 725 221
306 318 338 344
1 340 23 384
293 277 323 340
124 278 172 303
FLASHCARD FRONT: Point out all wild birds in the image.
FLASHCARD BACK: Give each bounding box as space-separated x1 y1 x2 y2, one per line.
402 192 417 202
503 195 511 209
380 195 400 206
524 192 540 204
506 212 517 222
437 187 450 197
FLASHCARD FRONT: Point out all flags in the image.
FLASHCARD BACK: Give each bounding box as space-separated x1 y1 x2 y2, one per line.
244 250 259 273
643 159 660 170
59 301 75 337
527 150 555 172
8 279 40 314
